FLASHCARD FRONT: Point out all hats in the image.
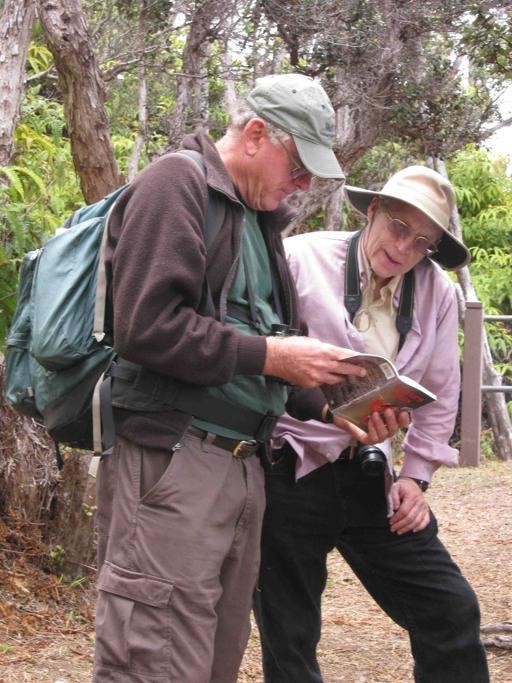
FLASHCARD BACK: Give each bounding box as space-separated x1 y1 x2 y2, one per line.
246 73 345 179
342 165 471 271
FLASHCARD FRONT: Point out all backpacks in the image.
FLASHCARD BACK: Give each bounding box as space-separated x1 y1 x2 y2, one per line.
5 149 227 449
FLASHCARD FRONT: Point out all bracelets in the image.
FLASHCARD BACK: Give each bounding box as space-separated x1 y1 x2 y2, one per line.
325 409 334 424
414 479 428 491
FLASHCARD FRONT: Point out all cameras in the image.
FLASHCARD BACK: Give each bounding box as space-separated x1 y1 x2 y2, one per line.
357 443 385 477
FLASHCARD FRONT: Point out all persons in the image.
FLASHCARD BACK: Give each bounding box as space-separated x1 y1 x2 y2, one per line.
91 72 366 683
252 165 490 683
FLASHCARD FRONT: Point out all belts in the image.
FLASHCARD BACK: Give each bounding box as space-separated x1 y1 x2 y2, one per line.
186 423 261 459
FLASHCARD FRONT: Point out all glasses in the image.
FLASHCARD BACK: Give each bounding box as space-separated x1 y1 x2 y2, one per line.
270 128 308 181
379 200 439 256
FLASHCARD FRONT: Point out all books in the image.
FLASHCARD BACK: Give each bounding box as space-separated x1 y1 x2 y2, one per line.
318 353 438 431
